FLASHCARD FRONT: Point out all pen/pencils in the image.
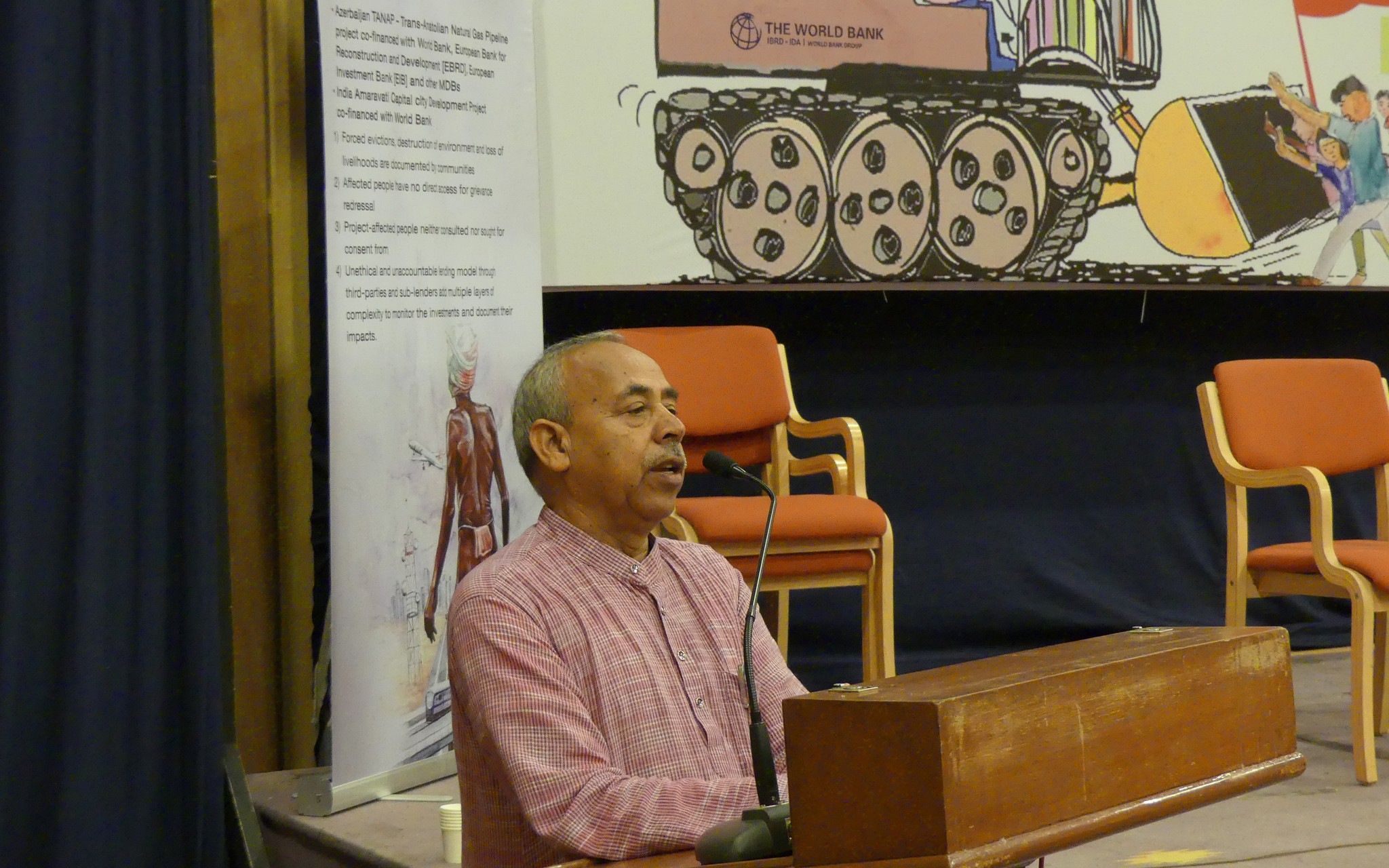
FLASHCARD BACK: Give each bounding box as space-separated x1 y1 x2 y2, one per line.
737 664 750 709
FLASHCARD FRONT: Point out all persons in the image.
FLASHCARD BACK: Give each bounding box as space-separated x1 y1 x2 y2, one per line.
446 333 809 868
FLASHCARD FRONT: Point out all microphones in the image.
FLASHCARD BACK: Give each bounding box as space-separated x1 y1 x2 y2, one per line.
693 452 792 866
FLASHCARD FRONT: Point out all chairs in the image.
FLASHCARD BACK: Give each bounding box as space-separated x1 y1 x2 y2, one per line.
1197 359 1389 784
606 325 894 682
